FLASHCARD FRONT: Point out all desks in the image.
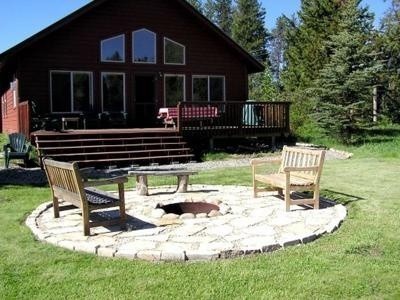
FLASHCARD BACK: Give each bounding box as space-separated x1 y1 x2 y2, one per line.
156 105 220 129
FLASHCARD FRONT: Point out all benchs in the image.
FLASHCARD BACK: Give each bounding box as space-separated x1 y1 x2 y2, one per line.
127 164 200 196
249 144 327 212
41 155 128 236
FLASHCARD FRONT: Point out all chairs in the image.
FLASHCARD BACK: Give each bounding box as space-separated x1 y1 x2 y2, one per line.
31 99 131 130
3 131 32 169
